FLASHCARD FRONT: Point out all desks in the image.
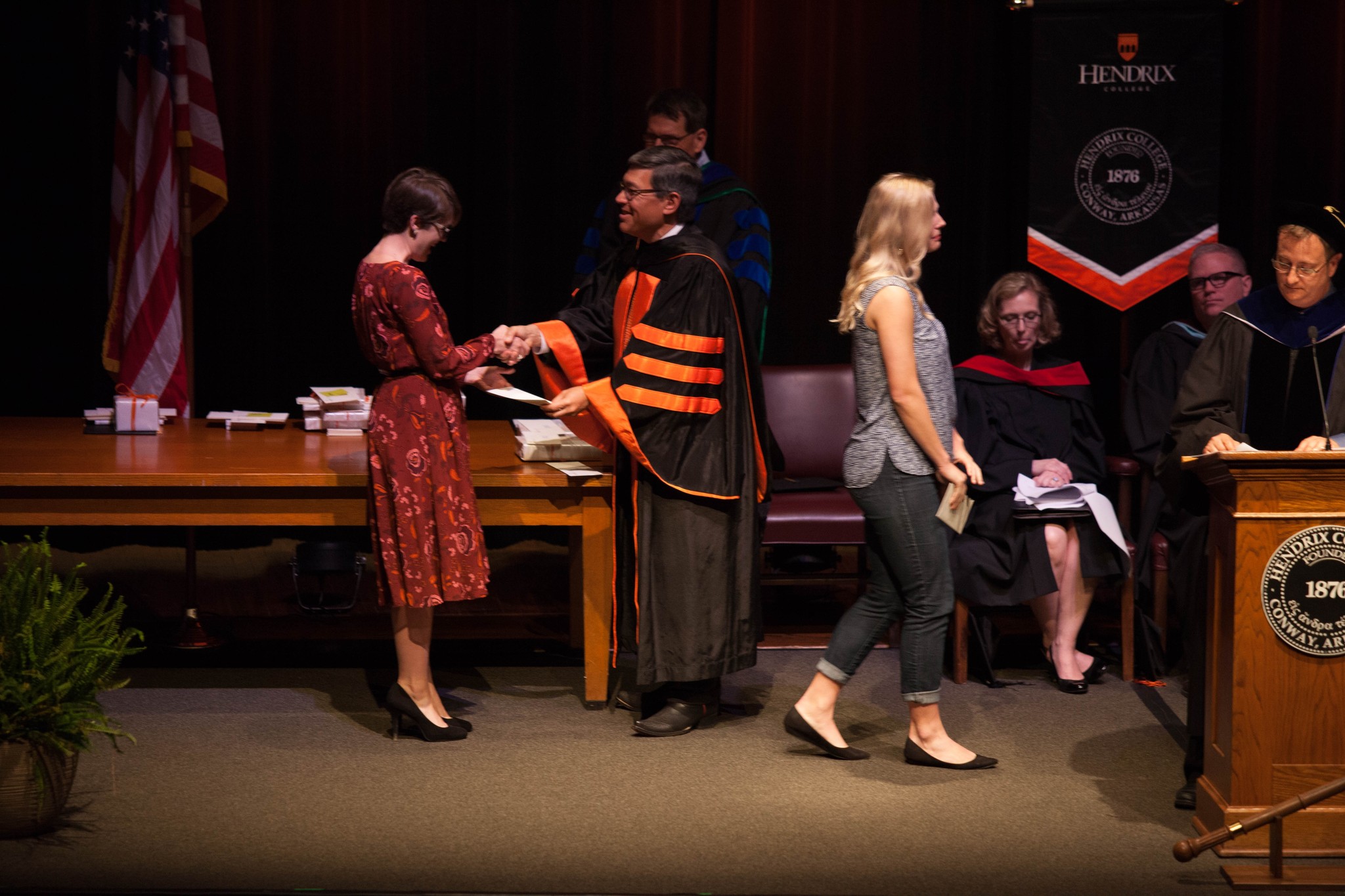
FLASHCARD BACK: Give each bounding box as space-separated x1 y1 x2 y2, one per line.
0 413 620 704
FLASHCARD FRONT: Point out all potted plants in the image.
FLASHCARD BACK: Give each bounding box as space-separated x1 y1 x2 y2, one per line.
0 524 148 842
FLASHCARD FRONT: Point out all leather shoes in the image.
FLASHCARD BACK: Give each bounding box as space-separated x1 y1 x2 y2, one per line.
615 690 667 712
631 702 718 737
1175 782 1197 807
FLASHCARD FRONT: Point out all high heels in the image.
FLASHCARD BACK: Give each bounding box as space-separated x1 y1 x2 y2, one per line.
1047 645 1088 693
441 715 472 732
1045 648 1108 682
384 682 467 742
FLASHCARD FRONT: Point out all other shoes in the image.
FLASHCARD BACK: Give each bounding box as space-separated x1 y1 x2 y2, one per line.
905 735 998 770
784 705 870 761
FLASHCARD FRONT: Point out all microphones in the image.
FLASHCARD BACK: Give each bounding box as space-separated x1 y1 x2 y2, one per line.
1306 325 1332 451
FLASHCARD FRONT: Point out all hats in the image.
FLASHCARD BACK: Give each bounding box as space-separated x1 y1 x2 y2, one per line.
1276 203 1345 254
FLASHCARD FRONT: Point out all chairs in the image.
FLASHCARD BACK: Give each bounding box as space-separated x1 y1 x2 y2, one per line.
948 451 1183 687
748 362 880 642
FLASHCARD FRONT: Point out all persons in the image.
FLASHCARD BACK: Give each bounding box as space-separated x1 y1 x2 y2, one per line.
784 171 997 770
948 274 1124 695
570 87 770 354
1169 201 1345 455
1117 242 1252 673
351 168 532 742
100 0 227 420
488 147 782 739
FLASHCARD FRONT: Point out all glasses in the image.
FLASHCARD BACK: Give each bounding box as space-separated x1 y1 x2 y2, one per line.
643 133 691 146
995 315 1041 330
619 181 671 201
1188 271 1243 291
432 222 452 239
1271 253 1328 279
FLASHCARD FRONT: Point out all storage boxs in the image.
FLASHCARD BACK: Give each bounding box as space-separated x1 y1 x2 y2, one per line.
115 394 159 431
